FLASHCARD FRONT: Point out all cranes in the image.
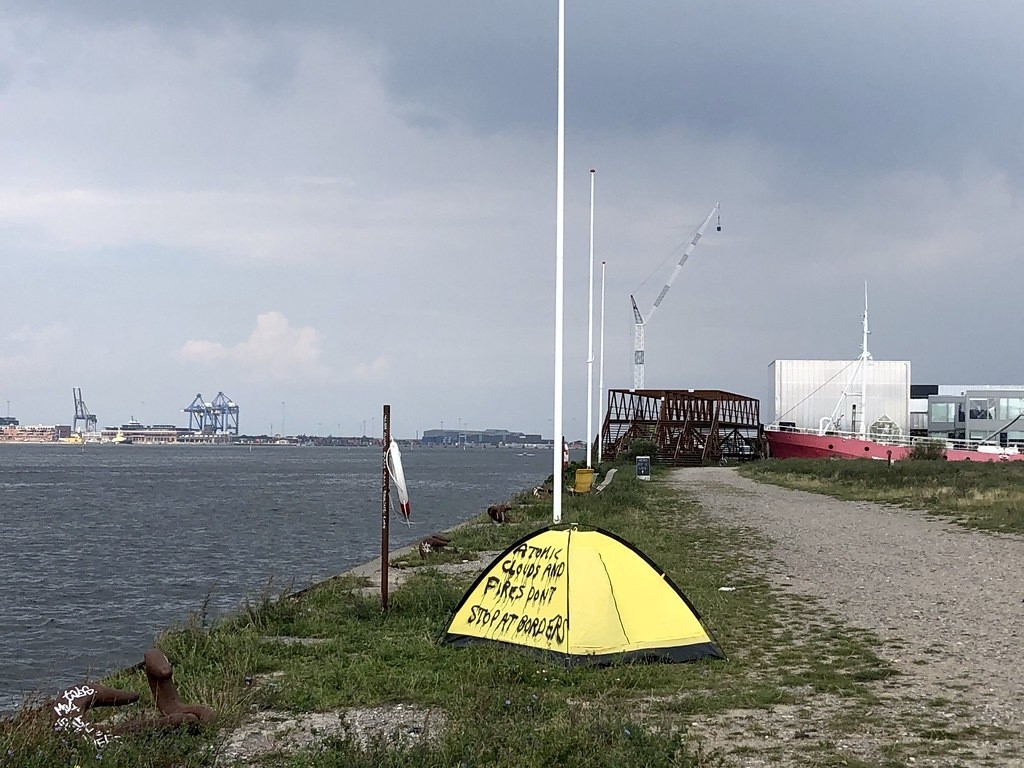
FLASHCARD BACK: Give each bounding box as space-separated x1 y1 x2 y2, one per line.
72 387 97 437
626 201 723 440
179 391 240 436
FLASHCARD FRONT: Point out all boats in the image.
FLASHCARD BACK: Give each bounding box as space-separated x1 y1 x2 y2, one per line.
764 279 1024 469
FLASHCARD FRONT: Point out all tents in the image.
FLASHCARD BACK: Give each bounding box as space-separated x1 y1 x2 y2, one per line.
438 522 722 664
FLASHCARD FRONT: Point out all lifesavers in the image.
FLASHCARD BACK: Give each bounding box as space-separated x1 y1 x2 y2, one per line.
388 439 411 519
